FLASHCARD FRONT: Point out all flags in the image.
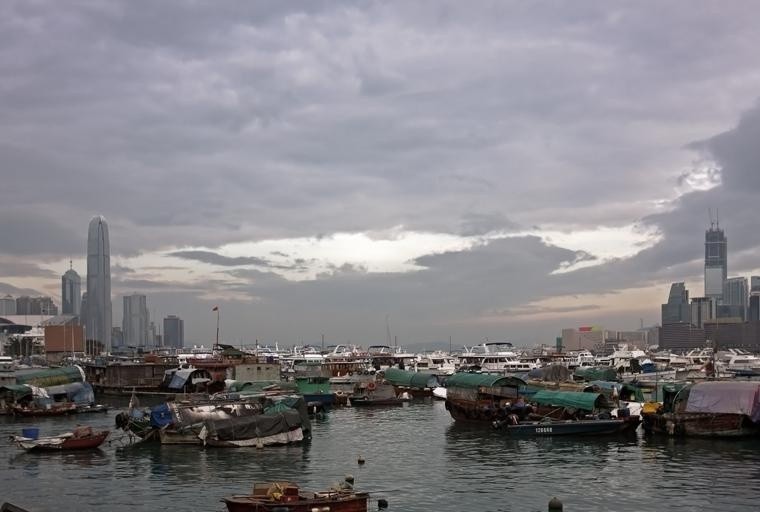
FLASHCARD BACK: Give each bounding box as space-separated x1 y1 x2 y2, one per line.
213 306 218 311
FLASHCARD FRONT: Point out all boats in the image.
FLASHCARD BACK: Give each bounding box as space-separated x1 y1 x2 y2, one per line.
220 492 369 512
